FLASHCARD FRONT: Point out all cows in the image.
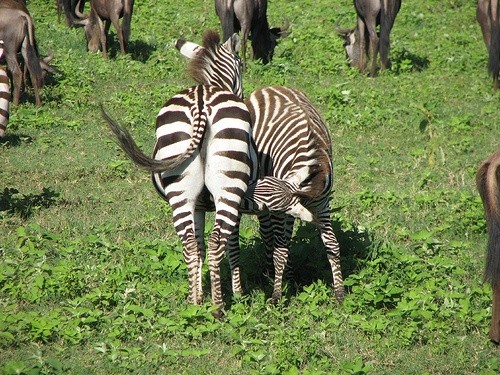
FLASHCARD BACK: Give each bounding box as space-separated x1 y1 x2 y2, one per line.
475 147 500 346
335 0 402 78
213 0 291 73
72 0 135 62
57 0 86 27
476 0 500 89
0 8 44 107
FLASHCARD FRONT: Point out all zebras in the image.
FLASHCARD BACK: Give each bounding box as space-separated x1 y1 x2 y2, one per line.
205 86 344 306
99 28 262 318
